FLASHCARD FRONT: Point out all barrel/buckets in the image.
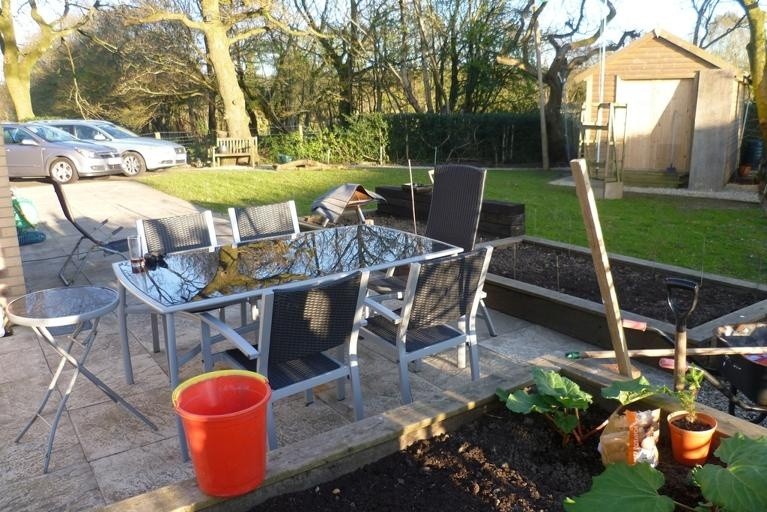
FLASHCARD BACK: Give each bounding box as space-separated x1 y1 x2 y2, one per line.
740 135 764 171
171 368 272 498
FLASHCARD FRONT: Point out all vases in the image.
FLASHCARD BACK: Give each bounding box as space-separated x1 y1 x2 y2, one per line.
278 153 294 163
739 164 753 176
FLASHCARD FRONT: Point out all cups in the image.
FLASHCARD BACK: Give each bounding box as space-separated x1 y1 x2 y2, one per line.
126 234 147 266
131 267 147 292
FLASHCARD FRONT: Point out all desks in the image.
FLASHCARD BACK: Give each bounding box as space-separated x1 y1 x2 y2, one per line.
4 283 163 477
108 218 466 469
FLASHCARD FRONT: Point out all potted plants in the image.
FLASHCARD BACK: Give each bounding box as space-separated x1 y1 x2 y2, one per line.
664 364 719 469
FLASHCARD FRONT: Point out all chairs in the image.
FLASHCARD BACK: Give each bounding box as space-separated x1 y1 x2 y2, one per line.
194 268 370 451
135 210 230 358
225 201 305 326
48 168 137 289
370 165 502 341
361 246 493 406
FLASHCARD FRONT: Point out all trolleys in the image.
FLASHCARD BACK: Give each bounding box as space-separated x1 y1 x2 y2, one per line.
620 317 767 424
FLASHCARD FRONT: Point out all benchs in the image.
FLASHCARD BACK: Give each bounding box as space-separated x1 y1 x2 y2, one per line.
208 135 261 166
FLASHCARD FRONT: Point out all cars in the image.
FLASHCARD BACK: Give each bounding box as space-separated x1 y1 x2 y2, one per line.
13 118 190 180
1 121 123 186
